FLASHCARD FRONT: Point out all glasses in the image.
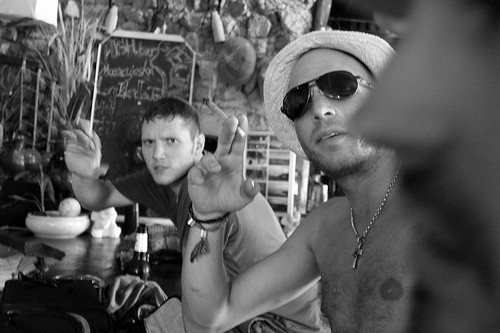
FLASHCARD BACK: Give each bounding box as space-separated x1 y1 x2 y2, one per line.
280 69 375 123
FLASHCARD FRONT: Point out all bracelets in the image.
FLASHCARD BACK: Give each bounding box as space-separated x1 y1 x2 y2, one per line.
188 201 231 262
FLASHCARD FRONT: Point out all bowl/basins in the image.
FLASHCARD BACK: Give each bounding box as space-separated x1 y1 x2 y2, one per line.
26 210 91 239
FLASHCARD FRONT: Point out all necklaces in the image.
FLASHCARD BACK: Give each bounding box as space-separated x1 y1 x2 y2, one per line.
349 155 403 270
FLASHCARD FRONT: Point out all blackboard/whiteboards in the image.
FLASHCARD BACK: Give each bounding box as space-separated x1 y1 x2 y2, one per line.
90 30 196 226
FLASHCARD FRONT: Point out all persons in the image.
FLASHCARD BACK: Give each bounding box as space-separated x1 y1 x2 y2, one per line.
62 95 322 333
181 0 500 333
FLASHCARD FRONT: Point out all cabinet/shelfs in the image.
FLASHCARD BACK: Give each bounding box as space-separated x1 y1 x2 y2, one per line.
243 129 311 236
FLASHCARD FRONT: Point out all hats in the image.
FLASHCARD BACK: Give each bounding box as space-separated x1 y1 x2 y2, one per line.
265 26 397 163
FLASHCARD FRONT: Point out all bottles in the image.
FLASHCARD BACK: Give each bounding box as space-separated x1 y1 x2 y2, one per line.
211 7 226 43
126 224 151 281
104 5 119 36
